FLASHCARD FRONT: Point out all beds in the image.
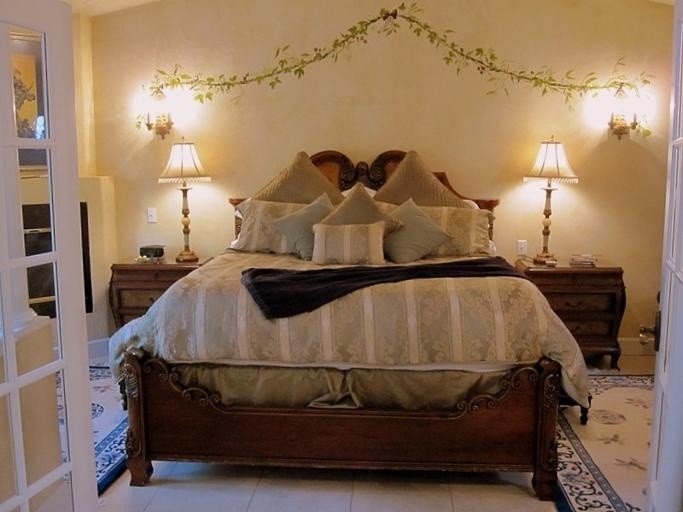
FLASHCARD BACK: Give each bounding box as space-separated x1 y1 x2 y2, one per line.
109 150 593 502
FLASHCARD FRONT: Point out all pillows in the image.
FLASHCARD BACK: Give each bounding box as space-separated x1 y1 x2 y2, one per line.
384 197 454 264
313 221 386 265
379 201 496 257
235 151 345 217
373 151 474 208
273 192 334 262
321 183 404 238
228 200 311 256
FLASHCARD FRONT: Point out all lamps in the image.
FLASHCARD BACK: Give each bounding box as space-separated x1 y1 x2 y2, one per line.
145 90 173 140
523 135 579 265
158 136 211 263
608 87 639 139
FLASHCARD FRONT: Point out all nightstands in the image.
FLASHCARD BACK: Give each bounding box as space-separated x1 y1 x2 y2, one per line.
109 258 202 330
515 259 626 371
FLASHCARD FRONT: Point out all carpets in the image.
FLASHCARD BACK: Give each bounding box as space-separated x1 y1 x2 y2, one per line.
57 365 129 497
555 368 655 511
